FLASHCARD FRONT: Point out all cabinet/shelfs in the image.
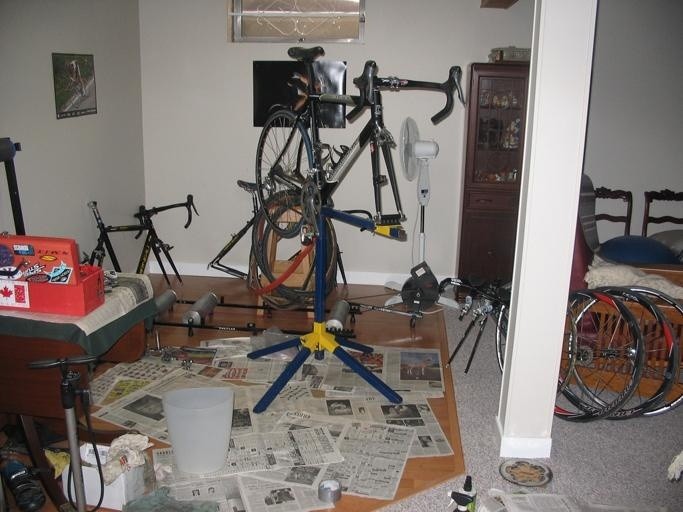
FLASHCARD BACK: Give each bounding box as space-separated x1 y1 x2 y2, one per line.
455 63 531 299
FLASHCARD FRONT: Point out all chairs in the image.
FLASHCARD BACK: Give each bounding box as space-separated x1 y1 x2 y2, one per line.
558 214 682 403
642 189 683 237
594 186 632 244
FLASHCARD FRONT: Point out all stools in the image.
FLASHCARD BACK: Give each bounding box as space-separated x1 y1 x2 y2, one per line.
257 204 320 318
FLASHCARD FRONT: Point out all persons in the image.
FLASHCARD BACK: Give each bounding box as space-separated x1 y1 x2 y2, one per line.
63 60 85 98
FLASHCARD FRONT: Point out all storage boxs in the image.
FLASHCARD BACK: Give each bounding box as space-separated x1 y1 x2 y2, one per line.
0 232 105 316
62 442 149 511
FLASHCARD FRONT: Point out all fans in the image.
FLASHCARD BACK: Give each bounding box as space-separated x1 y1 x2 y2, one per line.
384 116 459 309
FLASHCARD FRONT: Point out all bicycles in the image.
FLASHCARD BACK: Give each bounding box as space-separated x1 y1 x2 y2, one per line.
253 39 475 240
65 75 92 111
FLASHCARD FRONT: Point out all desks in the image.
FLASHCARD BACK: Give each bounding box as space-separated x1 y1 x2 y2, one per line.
0 270 158 512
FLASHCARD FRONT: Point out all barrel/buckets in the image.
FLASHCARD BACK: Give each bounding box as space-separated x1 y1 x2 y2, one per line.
162 386 234 473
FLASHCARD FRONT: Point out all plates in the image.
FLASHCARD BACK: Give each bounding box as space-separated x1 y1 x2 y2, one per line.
497 457 554 488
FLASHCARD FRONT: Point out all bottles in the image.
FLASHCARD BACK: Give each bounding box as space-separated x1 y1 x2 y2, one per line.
459 474 477 512
